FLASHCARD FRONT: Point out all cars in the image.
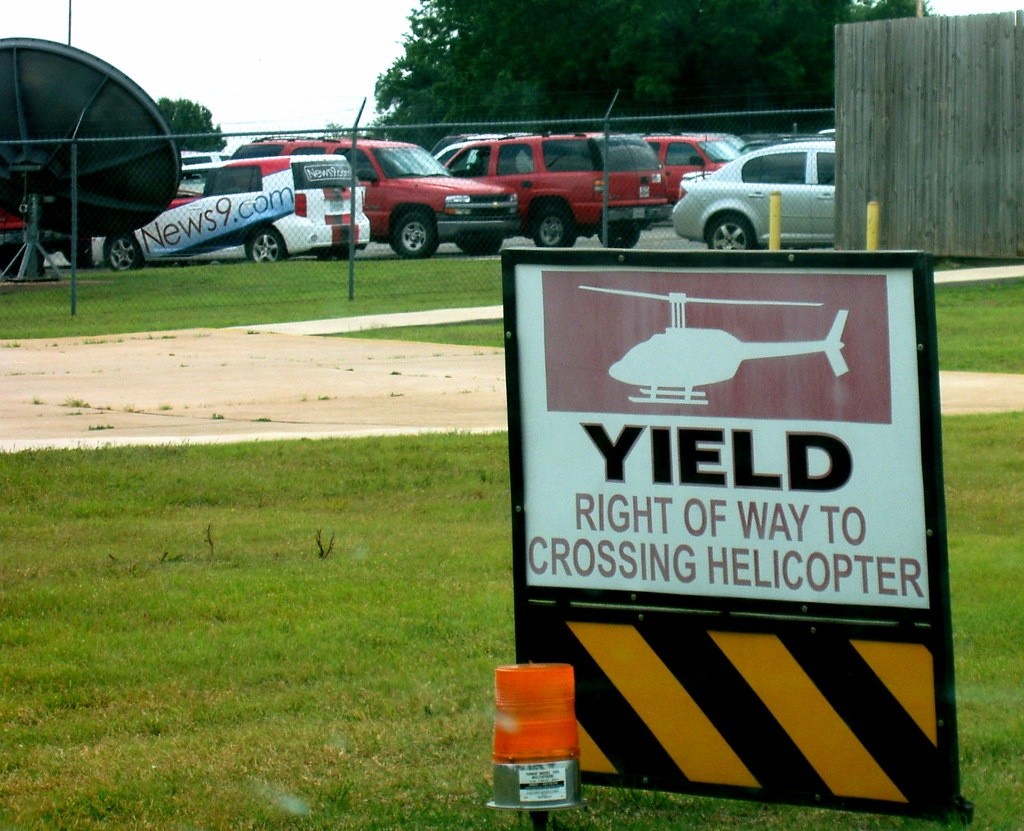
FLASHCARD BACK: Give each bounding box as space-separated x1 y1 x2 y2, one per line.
428 131 536 173
176 152 234 197
670 133 838 251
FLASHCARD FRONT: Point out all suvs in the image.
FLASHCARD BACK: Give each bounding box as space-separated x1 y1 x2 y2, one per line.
641 134 743 205
715 132 753 154
442 128 671 248
86 152 372 262
223 135 522 261
